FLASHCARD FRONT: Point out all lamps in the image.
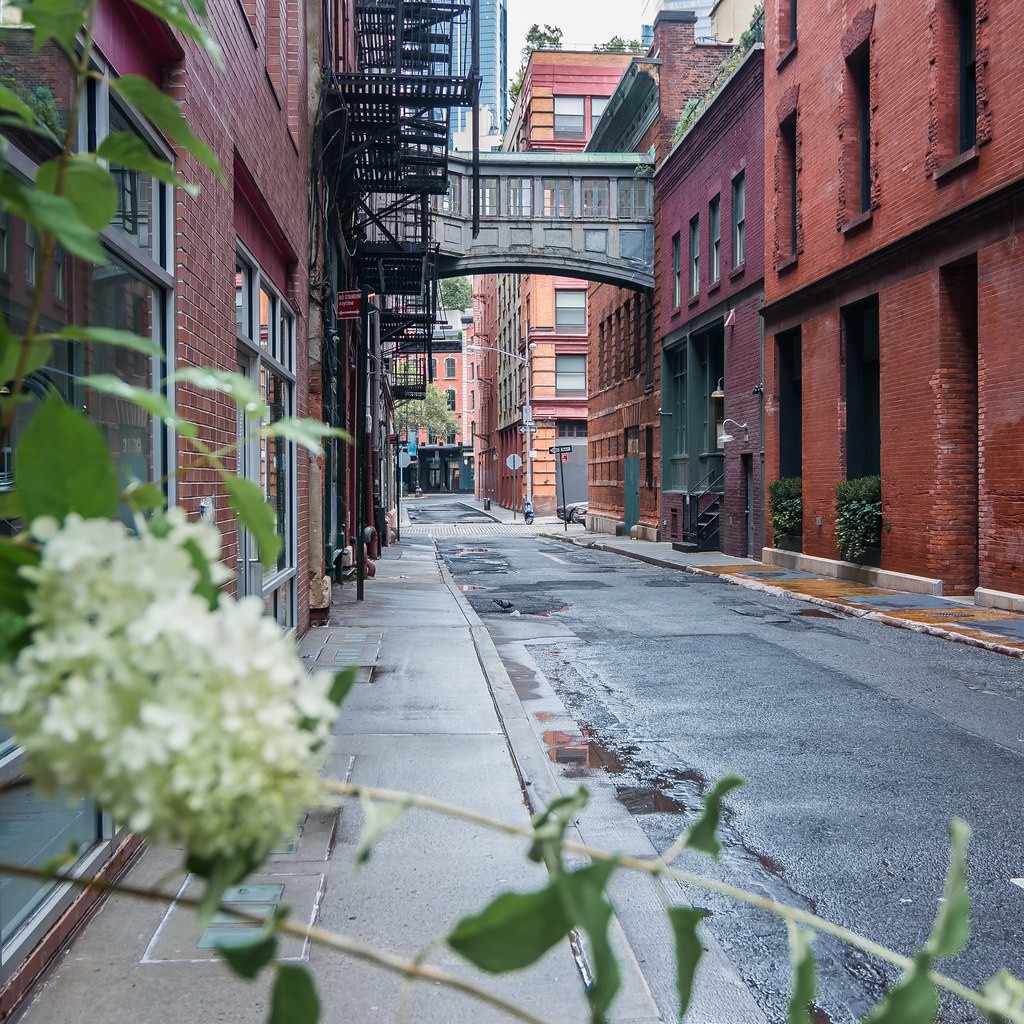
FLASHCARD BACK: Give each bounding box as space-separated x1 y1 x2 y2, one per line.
718 418 748 442
711 377 724 397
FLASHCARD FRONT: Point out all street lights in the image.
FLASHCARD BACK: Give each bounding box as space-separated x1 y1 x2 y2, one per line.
466 323 538 503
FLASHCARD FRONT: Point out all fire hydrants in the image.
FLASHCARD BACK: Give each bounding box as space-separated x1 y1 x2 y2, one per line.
415 485 420 497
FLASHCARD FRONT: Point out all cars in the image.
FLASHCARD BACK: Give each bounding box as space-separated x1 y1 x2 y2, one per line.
557 502 588 527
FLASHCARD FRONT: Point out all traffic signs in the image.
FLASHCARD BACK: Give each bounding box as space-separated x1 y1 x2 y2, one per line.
549 445 572 454
518 426 537 434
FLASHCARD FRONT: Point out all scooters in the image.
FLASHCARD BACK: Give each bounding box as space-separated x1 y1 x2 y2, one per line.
522 493 535 525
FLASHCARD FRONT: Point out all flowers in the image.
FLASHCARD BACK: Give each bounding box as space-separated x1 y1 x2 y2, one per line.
1 497 337 874
